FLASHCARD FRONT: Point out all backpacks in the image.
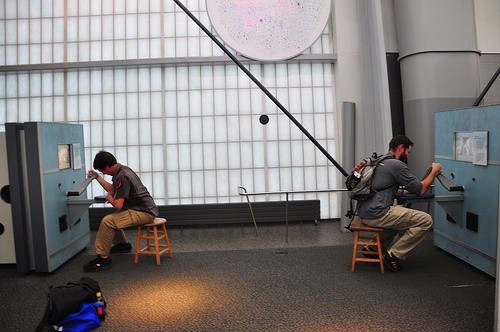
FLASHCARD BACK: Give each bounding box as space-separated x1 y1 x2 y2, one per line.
345 152 397 201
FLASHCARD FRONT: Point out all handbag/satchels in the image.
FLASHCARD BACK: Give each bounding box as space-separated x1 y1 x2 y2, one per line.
34 276 107 332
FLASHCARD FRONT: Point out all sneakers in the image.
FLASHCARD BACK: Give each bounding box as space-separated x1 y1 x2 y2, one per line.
362 246 378 259
82 255 112 272
382 249 402 272
110 242 132 253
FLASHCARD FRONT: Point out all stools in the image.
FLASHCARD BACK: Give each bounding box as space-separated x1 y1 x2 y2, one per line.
348 218 393 273
134 218 172 265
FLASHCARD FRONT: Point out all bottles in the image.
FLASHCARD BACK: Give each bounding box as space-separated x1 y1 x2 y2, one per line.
95 292 105 321
351 170 361 189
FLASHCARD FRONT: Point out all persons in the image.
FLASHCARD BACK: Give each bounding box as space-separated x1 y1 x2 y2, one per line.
83 151 159 272
356 135 442 272
